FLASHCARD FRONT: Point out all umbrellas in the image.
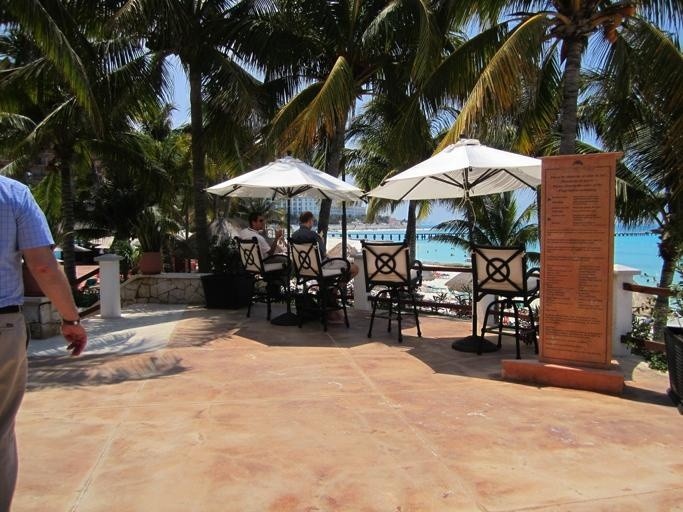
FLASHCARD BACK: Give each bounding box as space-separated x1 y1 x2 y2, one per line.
360 131 543 337
200 149 365 312
52 235 142 264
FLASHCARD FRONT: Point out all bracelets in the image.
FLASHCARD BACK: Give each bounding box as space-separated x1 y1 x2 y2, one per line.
61 317 81 326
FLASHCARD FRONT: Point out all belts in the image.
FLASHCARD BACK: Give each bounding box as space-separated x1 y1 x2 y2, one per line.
0 304 21 314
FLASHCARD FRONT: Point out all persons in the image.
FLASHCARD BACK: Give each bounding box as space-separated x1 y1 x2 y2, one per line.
0 175 87 512
237 210 289 299
290 211 360 311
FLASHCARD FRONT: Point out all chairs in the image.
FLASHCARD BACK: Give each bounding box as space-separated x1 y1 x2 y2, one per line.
231 232 352 334
356 238 422 343
469 244 541 361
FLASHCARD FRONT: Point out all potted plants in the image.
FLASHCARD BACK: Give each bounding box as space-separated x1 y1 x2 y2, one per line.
662 242 683 414
200 233 254 310
126 205 165 275
15 215 75 298
